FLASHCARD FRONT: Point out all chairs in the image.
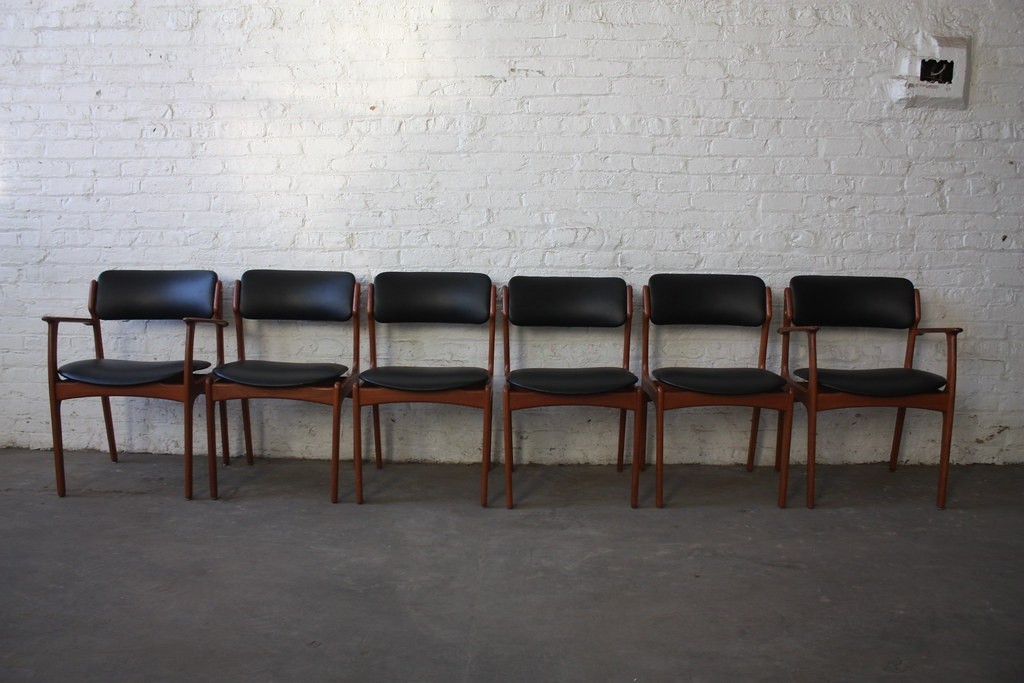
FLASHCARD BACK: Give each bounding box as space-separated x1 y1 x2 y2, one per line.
351 272 497 507
777 275 964 510
640 272 796 509
501 276 644 509
206 269 360 504
41 269 230 501
